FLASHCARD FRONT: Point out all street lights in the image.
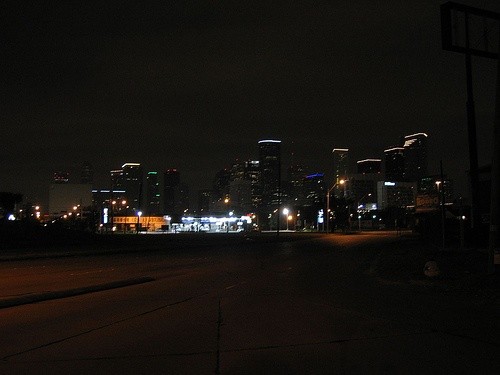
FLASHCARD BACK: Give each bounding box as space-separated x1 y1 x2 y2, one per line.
358 192 371 232
282 208 290 231
325 178 345 232
257 140 281 237
